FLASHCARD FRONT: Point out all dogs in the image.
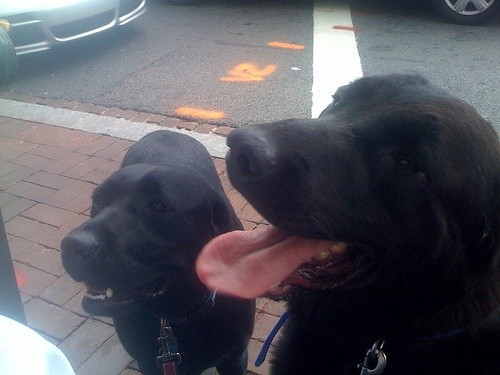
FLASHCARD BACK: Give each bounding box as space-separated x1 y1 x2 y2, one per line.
60 129 256 374
194 74 500 375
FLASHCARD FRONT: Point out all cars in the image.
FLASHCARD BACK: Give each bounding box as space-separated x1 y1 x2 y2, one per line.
0 1 147 87
